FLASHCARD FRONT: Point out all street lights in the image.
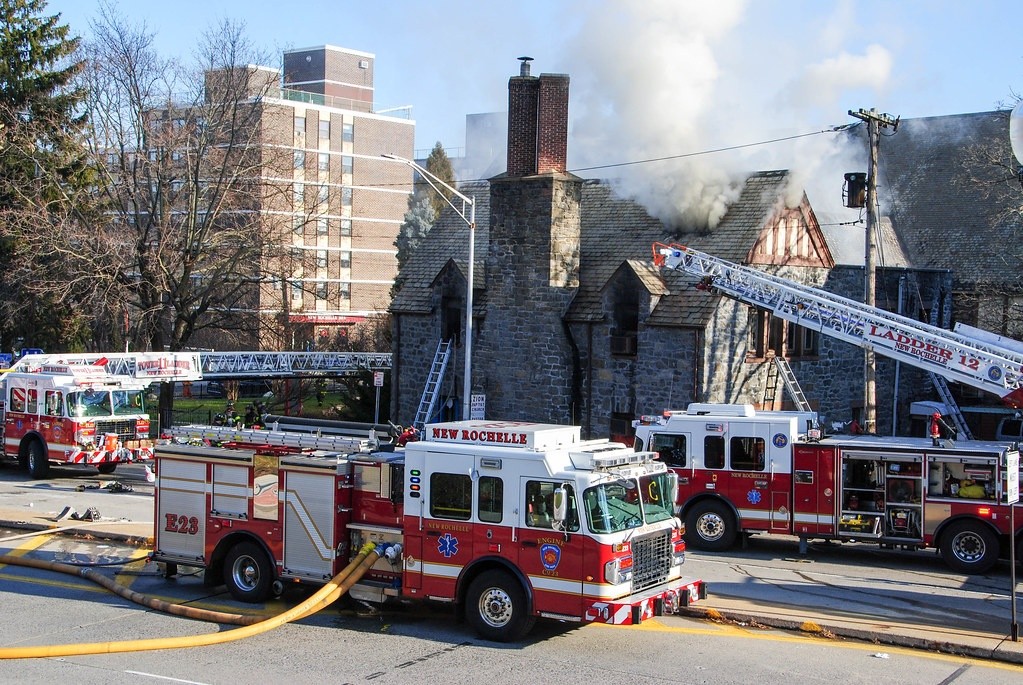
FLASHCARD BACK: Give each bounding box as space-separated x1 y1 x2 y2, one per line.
840 171 881 435
381 151 478 422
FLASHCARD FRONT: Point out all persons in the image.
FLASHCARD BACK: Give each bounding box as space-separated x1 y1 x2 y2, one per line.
956 479 988 499
211 399 271 432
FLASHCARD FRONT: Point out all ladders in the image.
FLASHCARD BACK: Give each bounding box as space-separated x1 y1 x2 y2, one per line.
413 337 453 440
929 369 976 441
772 355 819 430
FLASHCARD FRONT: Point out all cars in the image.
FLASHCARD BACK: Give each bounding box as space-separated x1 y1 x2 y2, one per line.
206 380 274 398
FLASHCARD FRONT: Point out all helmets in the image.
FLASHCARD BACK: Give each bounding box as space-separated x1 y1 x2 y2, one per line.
226 400 235 407
960 479 972 485
245 404 255 410
215 412 226 421
254 400 261 406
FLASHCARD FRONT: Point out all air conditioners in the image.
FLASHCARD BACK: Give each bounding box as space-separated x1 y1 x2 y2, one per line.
610 336 634 355
609 417 632 436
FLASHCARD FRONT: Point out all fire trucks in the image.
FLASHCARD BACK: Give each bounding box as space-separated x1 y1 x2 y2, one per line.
0 350 395 480
147 417 708 645
630 242 1022 577
